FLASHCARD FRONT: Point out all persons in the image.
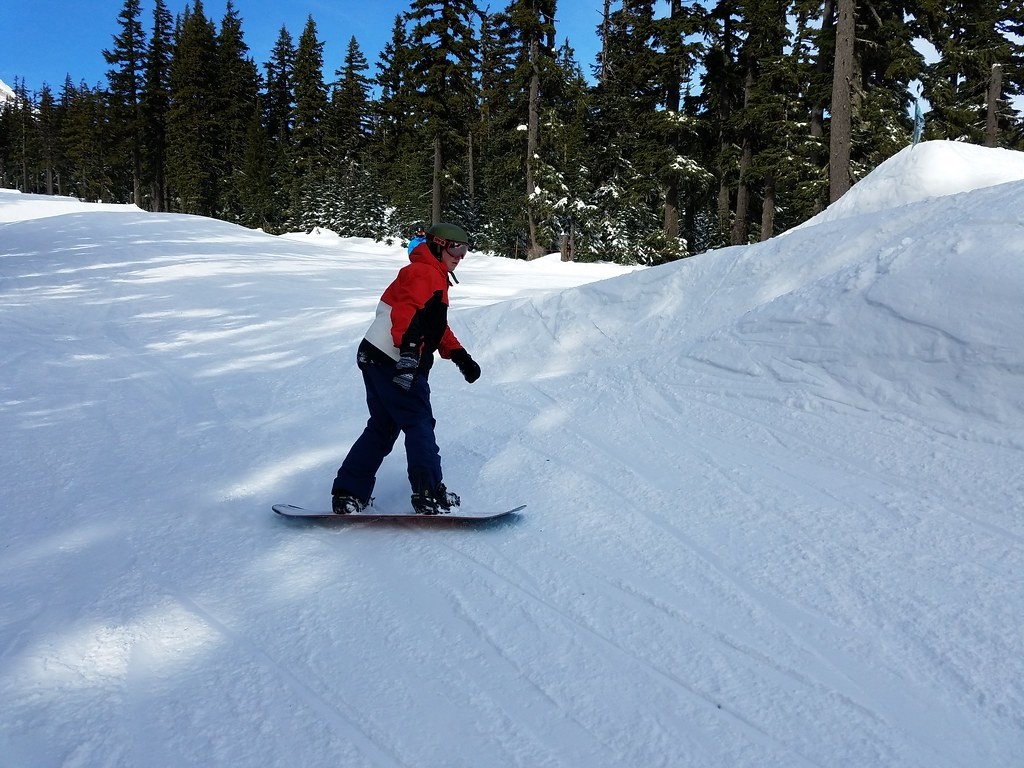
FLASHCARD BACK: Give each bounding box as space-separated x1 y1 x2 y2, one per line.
331 222 481 514
408 225 426 256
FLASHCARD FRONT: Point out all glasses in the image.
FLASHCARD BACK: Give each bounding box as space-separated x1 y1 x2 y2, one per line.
446 241 469 259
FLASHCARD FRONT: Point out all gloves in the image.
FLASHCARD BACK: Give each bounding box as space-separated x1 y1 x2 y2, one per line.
450 347 482 384
392 352 425 393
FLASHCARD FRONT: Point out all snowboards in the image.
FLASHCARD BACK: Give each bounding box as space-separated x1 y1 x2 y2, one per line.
272 504 526 519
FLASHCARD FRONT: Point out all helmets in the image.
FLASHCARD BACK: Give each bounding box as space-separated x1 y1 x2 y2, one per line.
426 223 468 262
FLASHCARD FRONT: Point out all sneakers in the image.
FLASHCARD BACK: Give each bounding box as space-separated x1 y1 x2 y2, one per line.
331 494 376 514
411 483 461 514
